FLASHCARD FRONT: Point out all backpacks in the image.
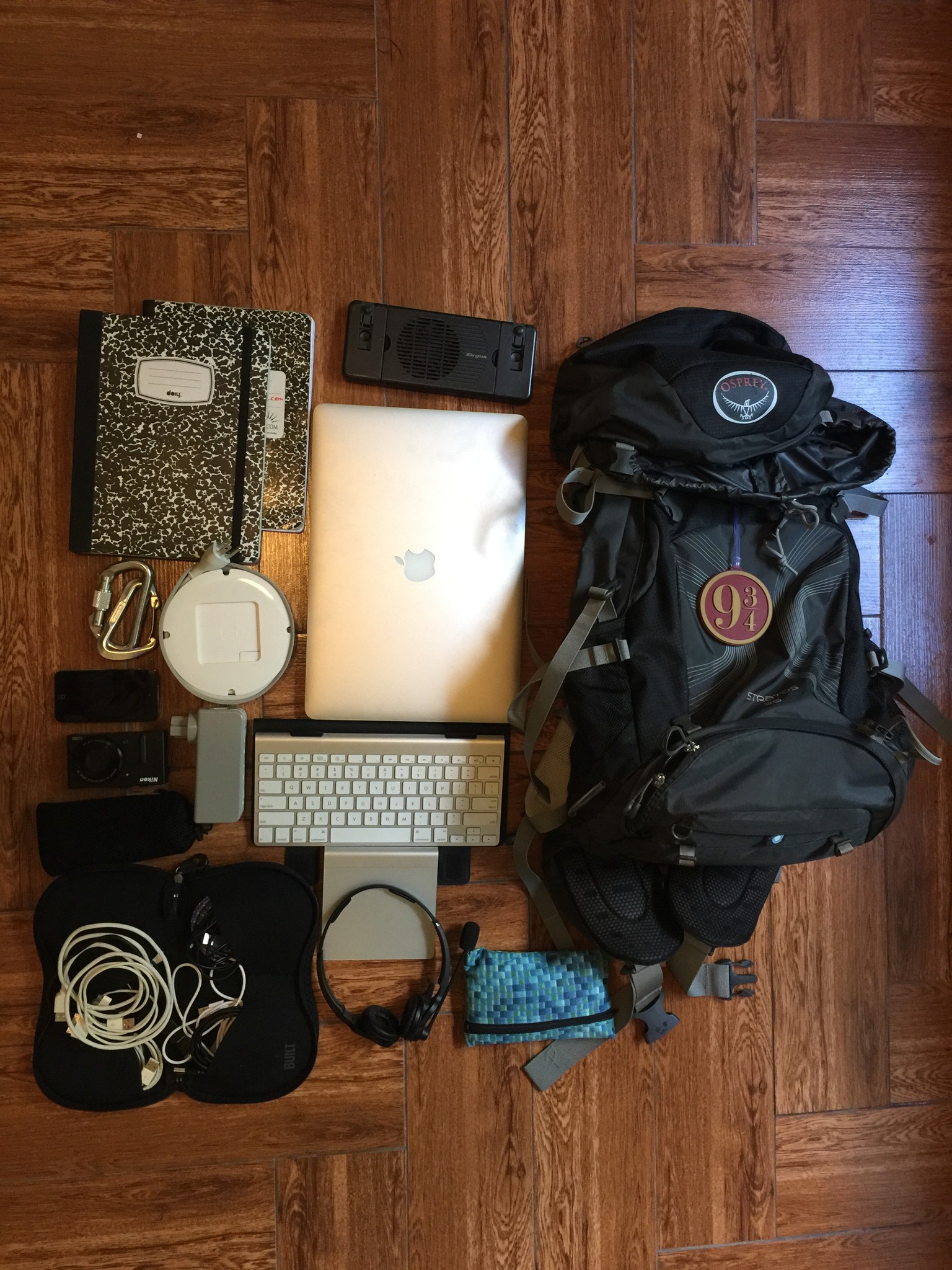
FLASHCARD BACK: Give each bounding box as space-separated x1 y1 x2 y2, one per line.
548 307 897 524
510 487 948 869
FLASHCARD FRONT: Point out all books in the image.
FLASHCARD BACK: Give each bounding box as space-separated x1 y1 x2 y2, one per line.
69 300 314 564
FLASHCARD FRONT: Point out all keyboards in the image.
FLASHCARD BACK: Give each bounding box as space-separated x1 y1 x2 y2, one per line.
251 718 509 850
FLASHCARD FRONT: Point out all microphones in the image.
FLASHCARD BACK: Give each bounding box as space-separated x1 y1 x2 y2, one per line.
420 921 480 1040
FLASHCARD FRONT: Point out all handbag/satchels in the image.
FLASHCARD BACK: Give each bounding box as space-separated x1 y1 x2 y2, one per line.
36 789 214 877
460 946 615 1046
31 853 319 1112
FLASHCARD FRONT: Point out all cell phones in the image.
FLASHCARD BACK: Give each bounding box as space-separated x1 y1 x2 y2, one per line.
54 667 161 723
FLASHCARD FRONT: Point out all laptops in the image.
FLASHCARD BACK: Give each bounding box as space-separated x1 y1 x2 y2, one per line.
302 404 528 727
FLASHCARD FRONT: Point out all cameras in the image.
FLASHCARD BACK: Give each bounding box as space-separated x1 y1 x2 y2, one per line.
67 730 170 789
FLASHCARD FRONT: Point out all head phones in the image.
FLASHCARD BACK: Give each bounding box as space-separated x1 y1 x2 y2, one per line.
316 885 451 1048
